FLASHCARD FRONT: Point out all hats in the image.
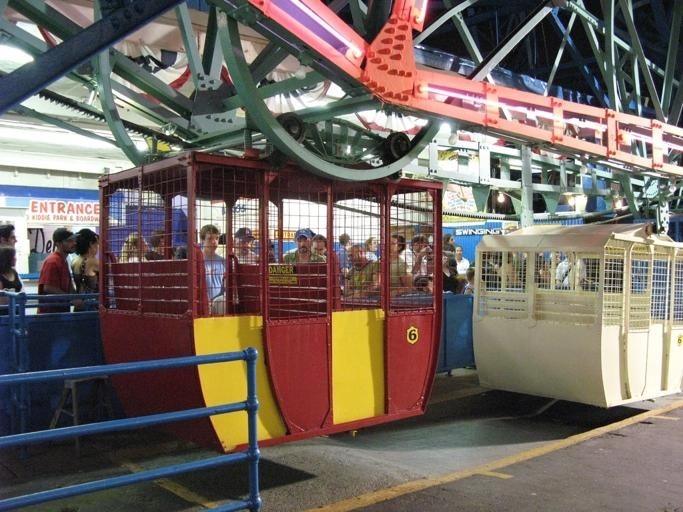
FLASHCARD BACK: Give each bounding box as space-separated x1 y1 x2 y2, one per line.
199 225 218 240
295 229 312 240
235 227 255 240
53 227 81 242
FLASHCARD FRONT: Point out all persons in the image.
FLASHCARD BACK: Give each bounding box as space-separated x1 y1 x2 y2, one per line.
199 225 474 316
37 227 186 316
1 225 22 317
481 251 586 291
